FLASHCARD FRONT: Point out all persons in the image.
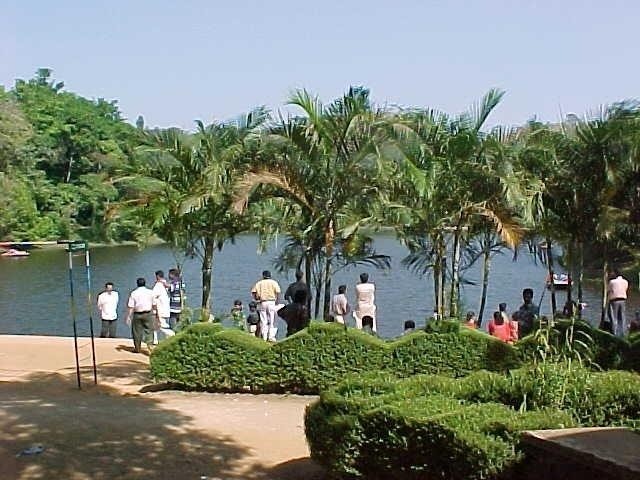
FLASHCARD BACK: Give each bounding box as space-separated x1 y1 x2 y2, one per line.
231 271 312 342
361 316 373 332
332 286 350 324
464 288 538 344
631 311 640 330
553 272 568 280
607 271 629 336
97 282 119 338
401 320 415 335
126 269 185 353
356 273 376 310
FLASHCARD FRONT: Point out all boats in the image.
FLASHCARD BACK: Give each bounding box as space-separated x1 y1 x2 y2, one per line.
1 249 30 256
545 271 573 290
64 244 85 253
